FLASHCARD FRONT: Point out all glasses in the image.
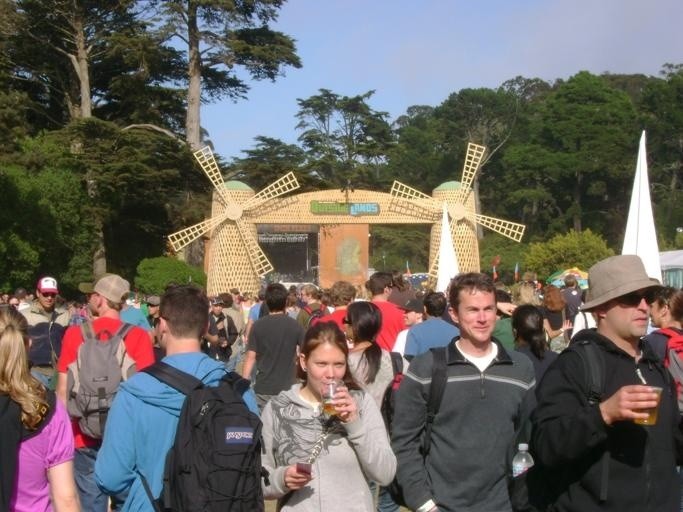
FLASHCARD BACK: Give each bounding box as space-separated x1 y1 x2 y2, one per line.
41 292 58 298
611 290 655 306
342 318 351 325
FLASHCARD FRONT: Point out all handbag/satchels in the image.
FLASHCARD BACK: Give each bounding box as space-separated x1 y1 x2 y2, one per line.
549 331 571 355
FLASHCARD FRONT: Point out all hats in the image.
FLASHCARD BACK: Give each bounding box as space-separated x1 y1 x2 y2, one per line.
14 288 26 298
77 273 131 304
579 255 667 312
211 297 224 305
37 276 60 294
397 299 424 314
143 296 161 306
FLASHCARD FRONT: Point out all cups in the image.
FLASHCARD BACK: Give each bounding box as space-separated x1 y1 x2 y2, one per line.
317 378 346 417
631 386 663 425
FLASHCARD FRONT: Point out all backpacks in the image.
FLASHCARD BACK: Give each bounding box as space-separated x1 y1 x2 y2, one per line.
132 362 270 511
64 318 134 441
303 305 326 330
653 329 683 415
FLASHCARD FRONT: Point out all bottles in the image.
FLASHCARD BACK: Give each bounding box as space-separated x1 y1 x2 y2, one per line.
510 442 536 479
